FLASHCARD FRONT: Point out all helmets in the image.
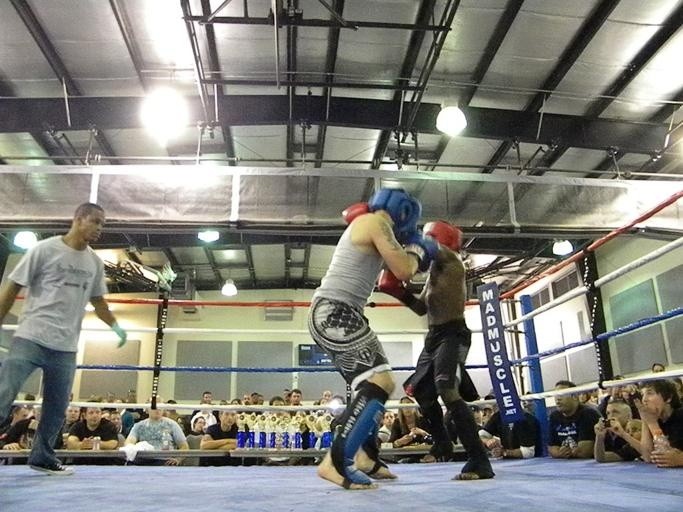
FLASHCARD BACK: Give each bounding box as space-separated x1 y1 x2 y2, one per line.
368 186 421 233
422 222 463 250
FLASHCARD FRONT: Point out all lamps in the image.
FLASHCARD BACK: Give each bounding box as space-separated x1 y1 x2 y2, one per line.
219 263 237 297
435 92 467 139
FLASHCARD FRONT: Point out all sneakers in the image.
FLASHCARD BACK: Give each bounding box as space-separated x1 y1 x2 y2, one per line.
30 460 73 477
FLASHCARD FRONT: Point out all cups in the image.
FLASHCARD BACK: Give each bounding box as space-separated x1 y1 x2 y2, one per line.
61 433 68 449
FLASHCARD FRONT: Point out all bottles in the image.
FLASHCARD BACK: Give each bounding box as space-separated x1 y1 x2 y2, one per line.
161 431 169 450
92 437 100 450
652 430 669 468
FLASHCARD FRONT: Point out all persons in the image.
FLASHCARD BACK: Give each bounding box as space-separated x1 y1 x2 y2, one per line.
0 391 190 466
0 203 128 477
189 388 344 466
377 397 436 463
443 388 543 460
342 197 495 482
307 187 429 493
545 362 683 467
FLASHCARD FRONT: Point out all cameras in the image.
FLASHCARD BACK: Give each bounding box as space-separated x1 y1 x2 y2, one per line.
602 418 615 428
409 432 417 439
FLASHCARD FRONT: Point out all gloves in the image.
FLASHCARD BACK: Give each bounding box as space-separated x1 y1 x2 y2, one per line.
378 269 407 299
341 203 368 224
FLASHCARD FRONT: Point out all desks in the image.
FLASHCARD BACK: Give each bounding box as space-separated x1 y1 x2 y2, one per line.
0 444 231 465
226 443 468 466
